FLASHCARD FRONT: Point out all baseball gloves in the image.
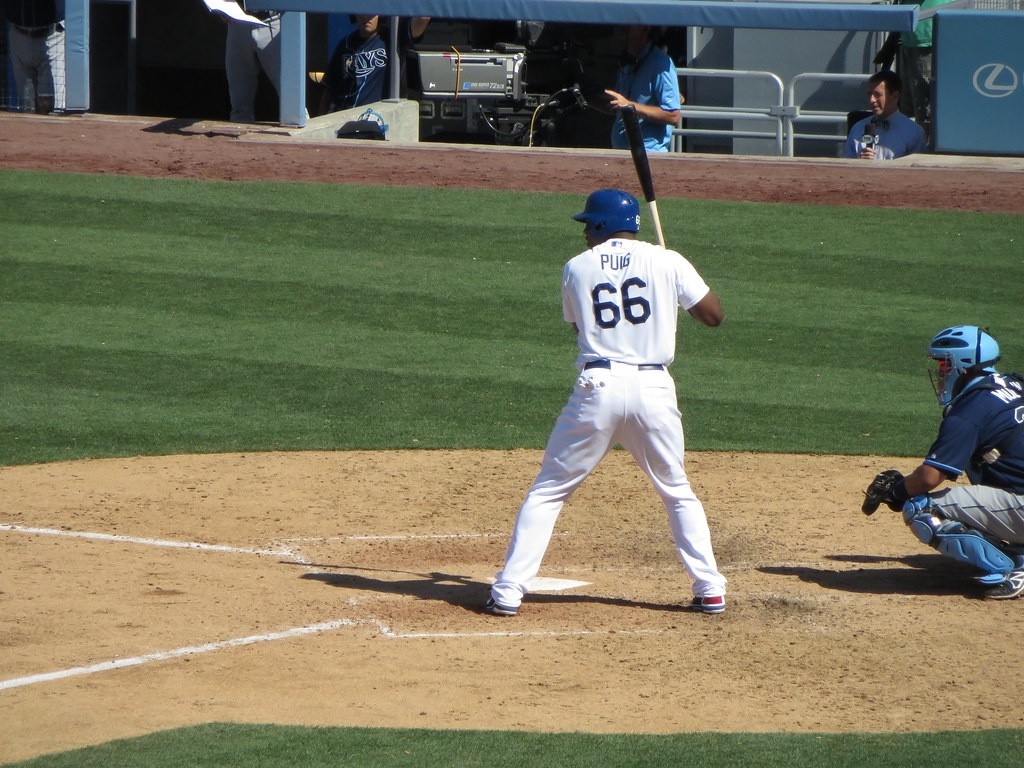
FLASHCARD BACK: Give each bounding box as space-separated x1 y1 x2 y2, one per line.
862 468 906 516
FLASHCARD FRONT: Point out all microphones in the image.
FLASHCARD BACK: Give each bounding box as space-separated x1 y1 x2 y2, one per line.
859 123 879 150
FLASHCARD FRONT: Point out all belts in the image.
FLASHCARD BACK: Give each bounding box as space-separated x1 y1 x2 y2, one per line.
250 10 278 20
13 24 62 38
583 360 665 371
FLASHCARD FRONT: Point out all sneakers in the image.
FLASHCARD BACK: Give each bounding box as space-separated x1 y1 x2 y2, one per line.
982 566 1024 599
691 596 726 613
485 597 522 615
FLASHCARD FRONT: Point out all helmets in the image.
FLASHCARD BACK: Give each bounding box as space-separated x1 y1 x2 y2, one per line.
571 189 641 248
926 325 1001 418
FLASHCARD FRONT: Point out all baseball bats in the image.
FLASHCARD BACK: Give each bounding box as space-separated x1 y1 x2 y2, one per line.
622 103 666 250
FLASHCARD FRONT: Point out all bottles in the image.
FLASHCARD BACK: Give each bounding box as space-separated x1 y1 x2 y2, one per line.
22 78 35 111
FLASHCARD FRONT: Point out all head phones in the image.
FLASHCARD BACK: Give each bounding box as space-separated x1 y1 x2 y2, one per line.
349 14 384 25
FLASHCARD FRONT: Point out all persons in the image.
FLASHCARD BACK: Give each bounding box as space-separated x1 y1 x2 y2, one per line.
841 71 928 158
861 325 1024 599
221 10 311 121
873 0 955 146
318 15 430 139
5 0 69 113
485 190 729 616
604 24 686 152
